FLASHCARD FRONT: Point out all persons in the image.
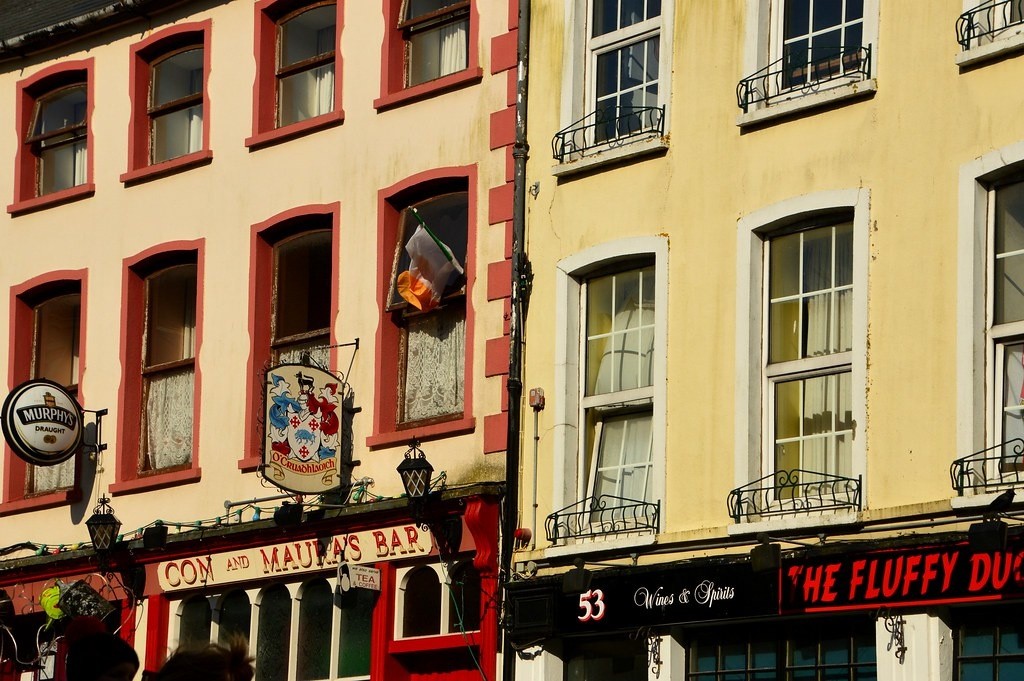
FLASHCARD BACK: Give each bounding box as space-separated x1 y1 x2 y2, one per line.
65 631 139 681
158 630 257 681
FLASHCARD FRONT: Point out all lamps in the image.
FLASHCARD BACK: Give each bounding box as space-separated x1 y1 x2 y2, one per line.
395 435 448 519
84 492 123 574
